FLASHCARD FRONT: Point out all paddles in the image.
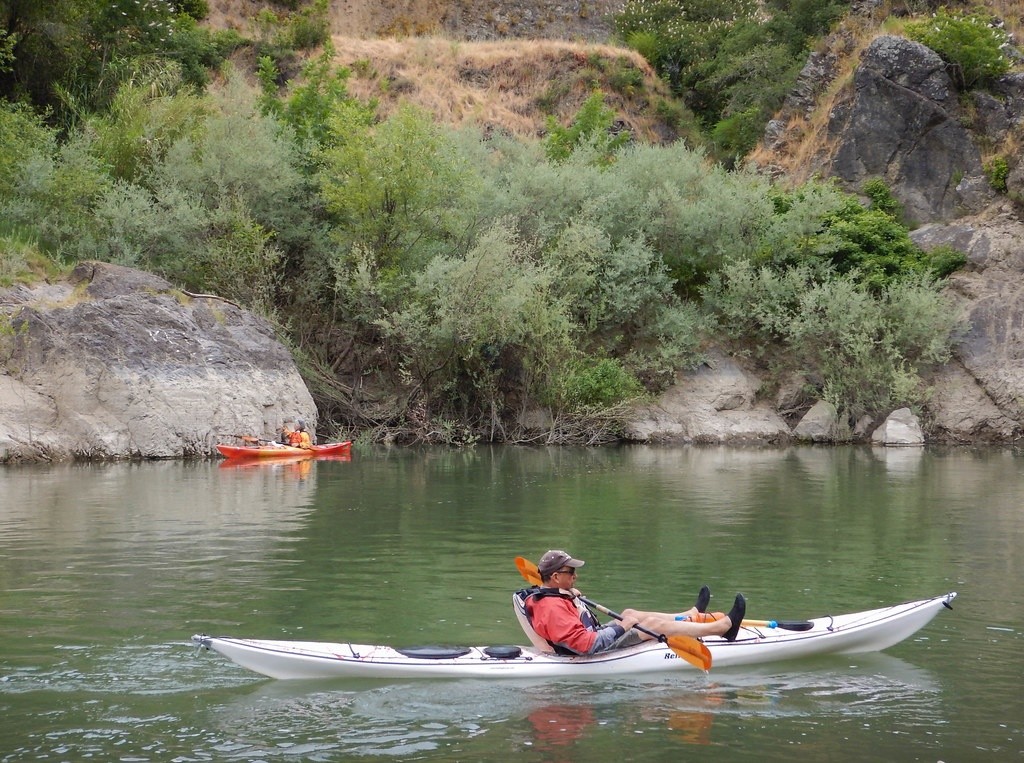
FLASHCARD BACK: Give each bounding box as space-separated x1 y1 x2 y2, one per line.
514 556 711 671
680 611 814 631
232 433 322 453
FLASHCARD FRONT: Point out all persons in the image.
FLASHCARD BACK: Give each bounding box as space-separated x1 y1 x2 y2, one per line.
282 419 313 449
521 549 746 657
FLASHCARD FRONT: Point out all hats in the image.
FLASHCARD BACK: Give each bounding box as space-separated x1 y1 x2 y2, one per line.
538 550 585 577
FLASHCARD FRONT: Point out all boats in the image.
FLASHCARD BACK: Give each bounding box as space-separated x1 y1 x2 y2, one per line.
193 592 959 680
216 441 353 459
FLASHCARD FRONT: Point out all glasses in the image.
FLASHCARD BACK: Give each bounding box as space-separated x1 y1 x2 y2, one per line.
557 567 575 575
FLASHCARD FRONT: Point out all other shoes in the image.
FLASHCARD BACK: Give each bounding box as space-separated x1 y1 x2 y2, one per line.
695 585 710 613
722 593 745 641
